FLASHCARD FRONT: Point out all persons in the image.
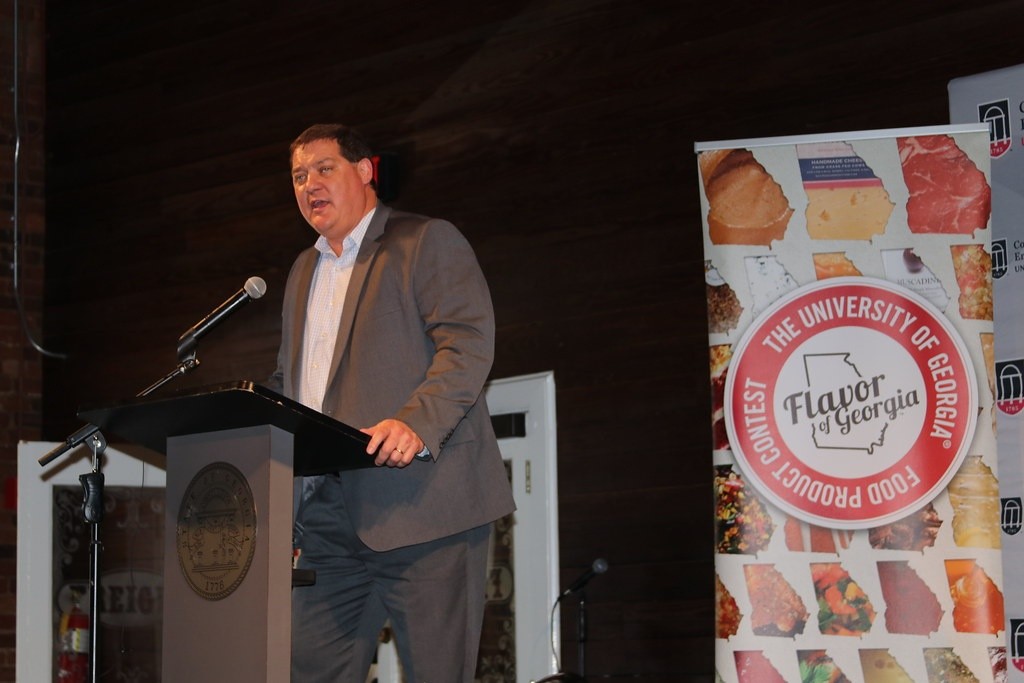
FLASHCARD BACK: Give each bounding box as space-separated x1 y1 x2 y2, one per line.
267 123 518 683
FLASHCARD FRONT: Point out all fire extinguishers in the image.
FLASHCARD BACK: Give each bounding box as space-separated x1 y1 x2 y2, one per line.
55 589 91 683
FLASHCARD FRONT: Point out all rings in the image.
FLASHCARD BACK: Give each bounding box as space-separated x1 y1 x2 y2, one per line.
395 448 405 455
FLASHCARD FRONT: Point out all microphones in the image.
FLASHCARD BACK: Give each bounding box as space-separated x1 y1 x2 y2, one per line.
558 559 608 601
177 277 266 346
